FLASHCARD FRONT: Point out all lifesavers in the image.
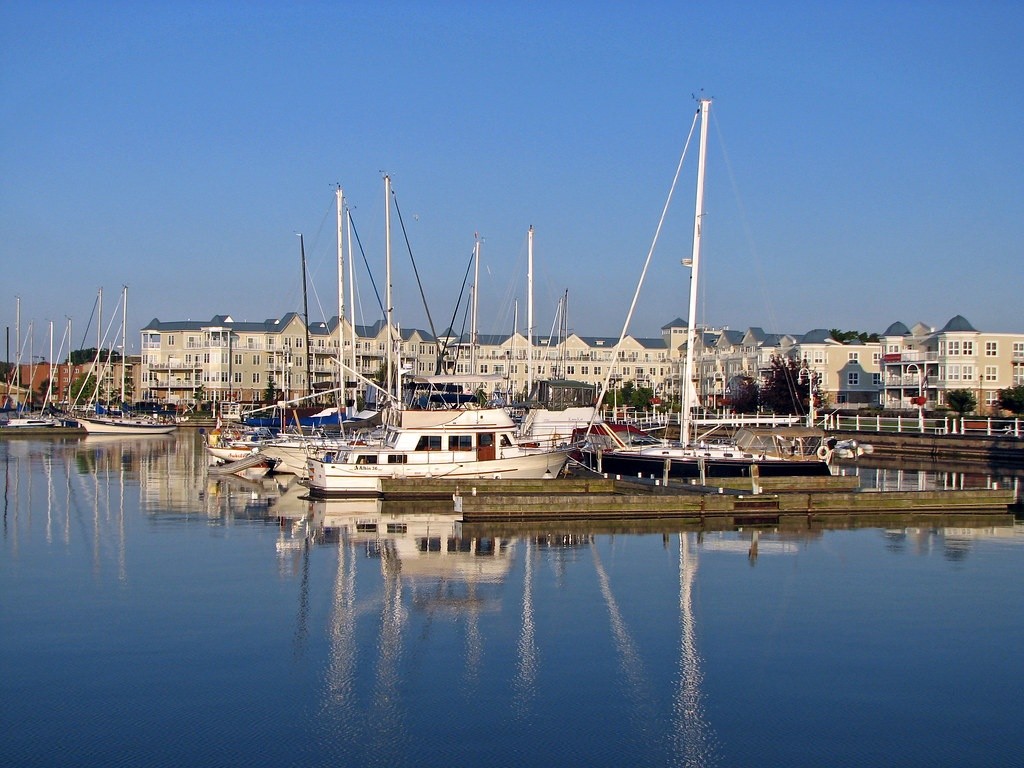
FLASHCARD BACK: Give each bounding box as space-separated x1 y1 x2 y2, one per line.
816 446 829 459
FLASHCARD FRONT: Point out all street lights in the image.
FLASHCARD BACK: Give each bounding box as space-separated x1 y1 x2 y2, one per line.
796 368 813 427
642 372 656 419
712 371 726 419
906 364 924 428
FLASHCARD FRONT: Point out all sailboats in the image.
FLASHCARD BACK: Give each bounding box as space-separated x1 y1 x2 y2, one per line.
0 281 181 436
201 170 611 505
576 98 838 476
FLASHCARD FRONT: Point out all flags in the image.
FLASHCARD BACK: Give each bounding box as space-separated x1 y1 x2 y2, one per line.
216 410 222 430
